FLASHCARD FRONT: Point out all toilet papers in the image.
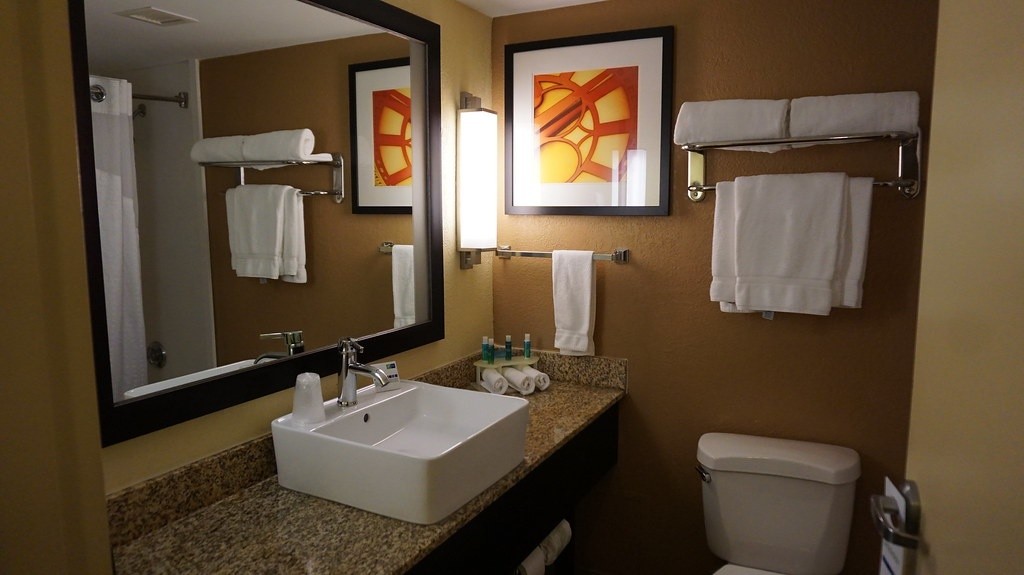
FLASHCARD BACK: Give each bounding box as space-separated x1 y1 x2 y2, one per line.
519 517 573 575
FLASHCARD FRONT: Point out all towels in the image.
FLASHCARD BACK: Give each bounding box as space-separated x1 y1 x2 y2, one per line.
480 368 548 394
190 134 245 166
391 244 415 329
787 91 921 145
225 184 308 283
673 96 789 153
242 127 333 169
707 172 877 315
552 250 597 356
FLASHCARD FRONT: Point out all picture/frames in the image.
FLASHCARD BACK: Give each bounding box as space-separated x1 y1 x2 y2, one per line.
502 24 674 218
346 57 415 215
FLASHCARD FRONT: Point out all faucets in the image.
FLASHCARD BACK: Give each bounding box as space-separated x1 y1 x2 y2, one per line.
253 330 304 366
336 336 391 406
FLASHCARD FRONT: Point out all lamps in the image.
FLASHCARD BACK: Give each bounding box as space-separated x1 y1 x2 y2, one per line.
456 91 498 269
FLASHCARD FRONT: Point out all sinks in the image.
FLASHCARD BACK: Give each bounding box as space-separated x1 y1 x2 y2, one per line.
124 356 278 401
269 378 529 525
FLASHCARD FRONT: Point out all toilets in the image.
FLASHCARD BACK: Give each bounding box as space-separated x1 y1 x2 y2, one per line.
695 433 861 574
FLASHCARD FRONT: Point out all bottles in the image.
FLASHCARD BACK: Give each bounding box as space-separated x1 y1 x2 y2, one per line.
481 336 488 362
488 339 495 365
506 335 511 360
524 334 530 359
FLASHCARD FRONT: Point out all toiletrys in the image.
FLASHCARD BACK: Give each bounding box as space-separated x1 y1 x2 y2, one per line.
482 335 489 362
488 338 495 364
505 335 512 361
524 333 531 358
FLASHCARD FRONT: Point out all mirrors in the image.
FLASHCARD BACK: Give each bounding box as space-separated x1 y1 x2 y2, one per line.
66 0 446 451
